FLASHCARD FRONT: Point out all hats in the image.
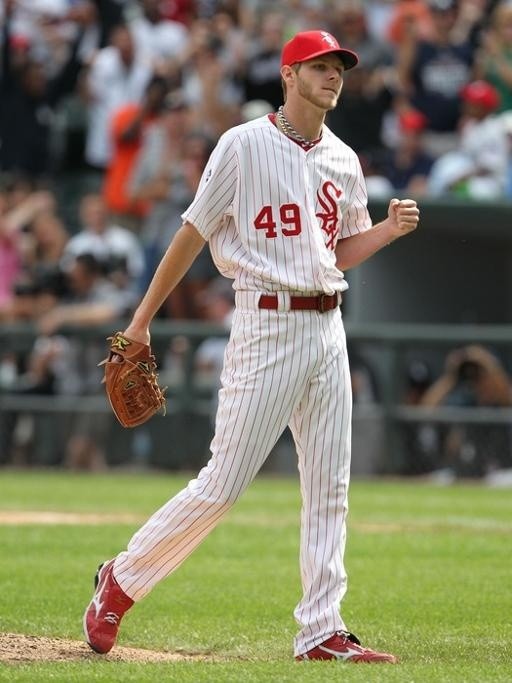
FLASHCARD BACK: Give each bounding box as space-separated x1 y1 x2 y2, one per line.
281 30 359 71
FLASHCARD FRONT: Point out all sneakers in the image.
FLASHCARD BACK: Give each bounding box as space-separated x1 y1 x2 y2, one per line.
295 631 396 663
83 558 134 654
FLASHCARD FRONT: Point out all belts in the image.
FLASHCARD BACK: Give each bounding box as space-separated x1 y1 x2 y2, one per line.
259 291 337 313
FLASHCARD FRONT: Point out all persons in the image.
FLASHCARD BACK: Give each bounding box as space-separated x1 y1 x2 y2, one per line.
1 0 512 482
82 30 421 662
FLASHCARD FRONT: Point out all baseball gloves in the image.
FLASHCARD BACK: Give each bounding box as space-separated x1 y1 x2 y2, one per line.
104 332 165 428
